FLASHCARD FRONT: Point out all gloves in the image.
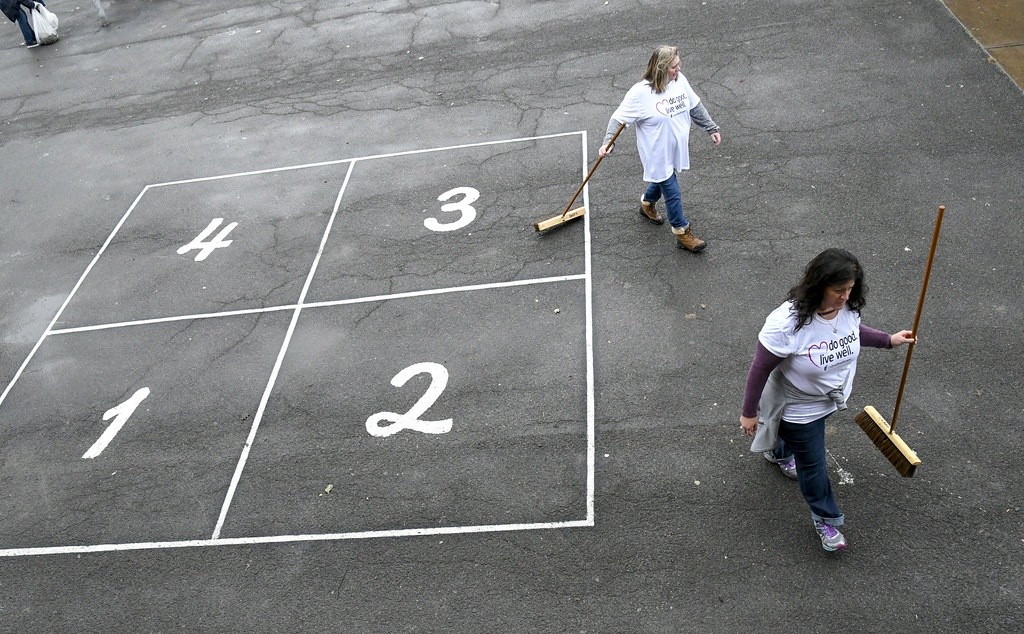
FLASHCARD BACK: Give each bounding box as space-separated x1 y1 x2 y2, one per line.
32 1 39 10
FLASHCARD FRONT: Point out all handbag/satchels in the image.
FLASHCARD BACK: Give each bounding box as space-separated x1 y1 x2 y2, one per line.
31 2 60 47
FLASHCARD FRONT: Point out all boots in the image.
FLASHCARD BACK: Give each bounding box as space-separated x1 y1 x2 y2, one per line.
671 223 708 252
639 192 665 225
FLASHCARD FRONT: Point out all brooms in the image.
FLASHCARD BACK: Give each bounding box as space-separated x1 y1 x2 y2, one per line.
532 120 627 234
853 202 946 477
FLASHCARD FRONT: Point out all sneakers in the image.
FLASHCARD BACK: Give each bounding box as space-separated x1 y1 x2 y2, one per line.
25 40 41 49
760 446 799 481
814 516 849 553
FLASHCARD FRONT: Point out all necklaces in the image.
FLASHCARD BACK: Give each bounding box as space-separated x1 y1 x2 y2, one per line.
817 309 839 333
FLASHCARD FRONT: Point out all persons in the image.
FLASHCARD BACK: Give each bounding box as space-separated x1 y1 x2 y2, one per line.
598 45 722 253
739 247 918 555
0 0 45 48
